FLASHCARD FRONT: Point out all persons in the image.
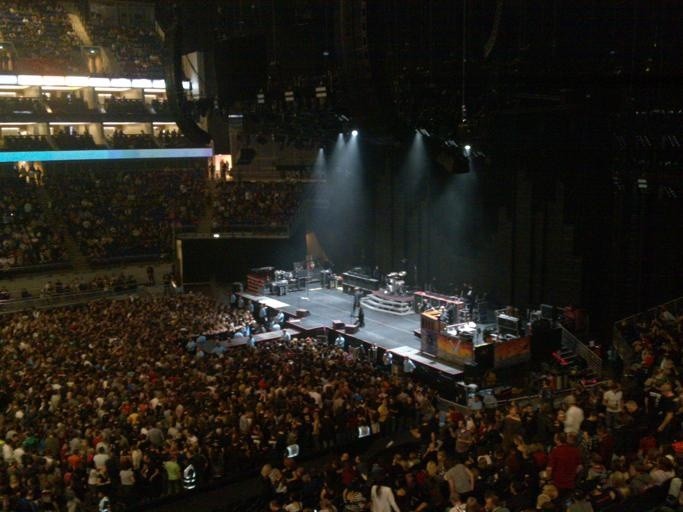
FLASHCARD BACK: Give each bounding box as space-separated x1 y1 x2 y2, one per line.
0 0 188 149
2 158 683 512
